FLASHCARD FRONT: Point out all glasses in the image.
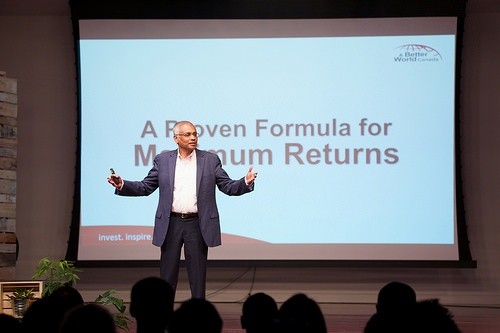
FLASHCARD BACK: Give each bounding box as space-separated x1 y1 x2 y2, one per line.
175 132 199 137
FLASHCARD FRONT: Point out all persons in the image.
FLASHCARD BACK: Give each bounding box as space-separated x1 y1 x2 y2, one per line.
0 277 463 333
107 121 258 299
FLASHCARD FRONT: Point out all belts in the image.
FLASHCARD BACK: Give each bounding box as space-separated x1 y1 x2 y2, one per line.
170 211 198 218
